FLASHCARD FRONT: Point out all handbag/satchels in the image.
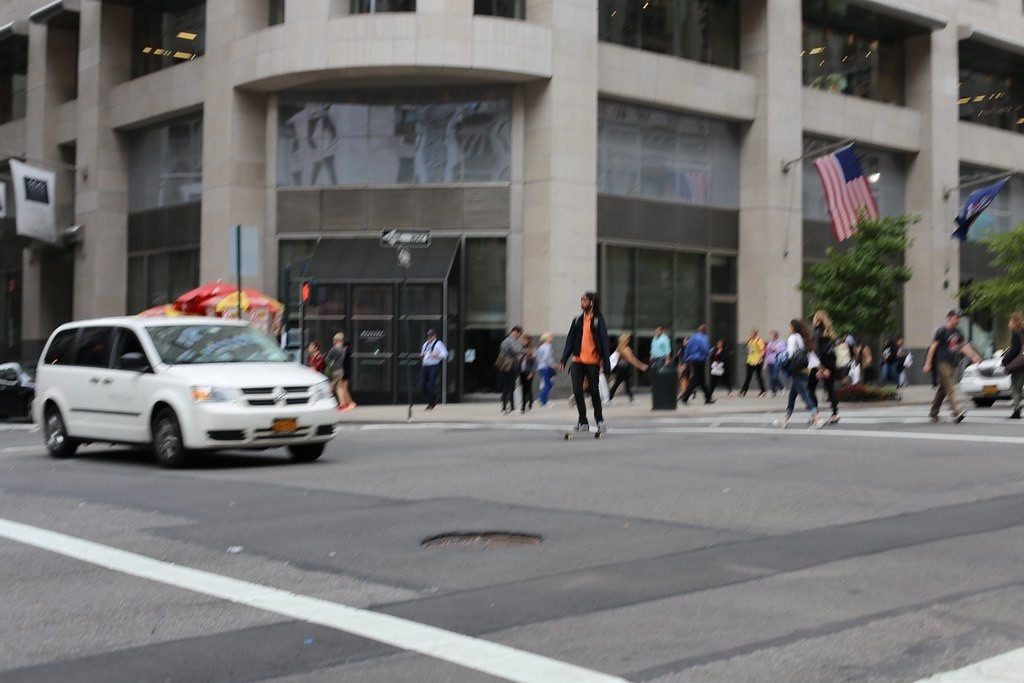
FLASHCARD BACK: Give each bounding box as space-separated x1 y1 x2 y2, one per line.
599 373 610 404
324 359 335 376
788 333 809 371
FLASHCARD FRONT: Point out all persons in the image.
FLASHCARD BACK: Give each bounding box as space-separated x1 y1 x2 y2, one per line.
763 330 787 399
560 291 611 432
306 333 356 413
498 326 536 414
923 310 981 424
738 327 767 398
875 336 912 388
670 324 736 404
533 333 557 408
774 309 871 428
648 325 672 377
608 334 647 404
419 329 450 409
1001 313 1024 418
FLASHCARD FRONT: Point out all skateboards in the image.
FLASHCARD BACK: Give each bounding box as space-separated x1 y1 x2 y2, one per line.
552 428 612 441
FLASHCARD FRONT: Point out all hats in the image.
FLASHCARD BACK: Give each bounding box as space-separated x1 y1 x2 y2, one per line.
427 328 435 335
538 331 550 342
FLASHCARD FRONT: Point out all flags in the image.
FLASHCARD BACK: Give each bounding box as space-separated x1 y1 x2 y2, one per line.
814 142 879 243
949 177 1010 241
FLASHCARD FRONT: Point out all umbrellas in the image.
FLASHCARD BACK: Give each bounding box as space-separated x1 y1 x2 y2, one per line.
131 282 285 319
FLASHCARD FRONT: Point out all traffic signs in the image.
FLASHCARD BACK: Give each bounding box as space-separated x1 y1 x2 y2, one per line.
380 226 432 251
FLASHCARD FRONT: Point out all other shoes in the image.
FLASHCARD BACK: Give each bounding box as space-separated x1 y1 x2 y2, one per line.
597 422 606 432
813 418 825 428
337 403 347 412
574 423 589 431
828 414 840 425
952 408 966 423
345 402 356 411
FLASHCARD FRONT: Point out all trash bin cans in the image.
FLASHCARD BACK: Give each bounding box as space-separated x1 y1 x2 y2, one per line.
651 367 679 410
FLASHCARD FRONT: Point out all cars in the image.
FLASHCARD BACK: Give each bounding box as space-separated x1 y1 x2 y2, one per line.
0 361 37 423
959 349 1012 408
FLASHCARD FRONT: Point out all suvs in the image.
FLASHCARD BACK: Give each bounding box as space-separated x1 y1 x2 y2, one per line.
31 314 341 467
